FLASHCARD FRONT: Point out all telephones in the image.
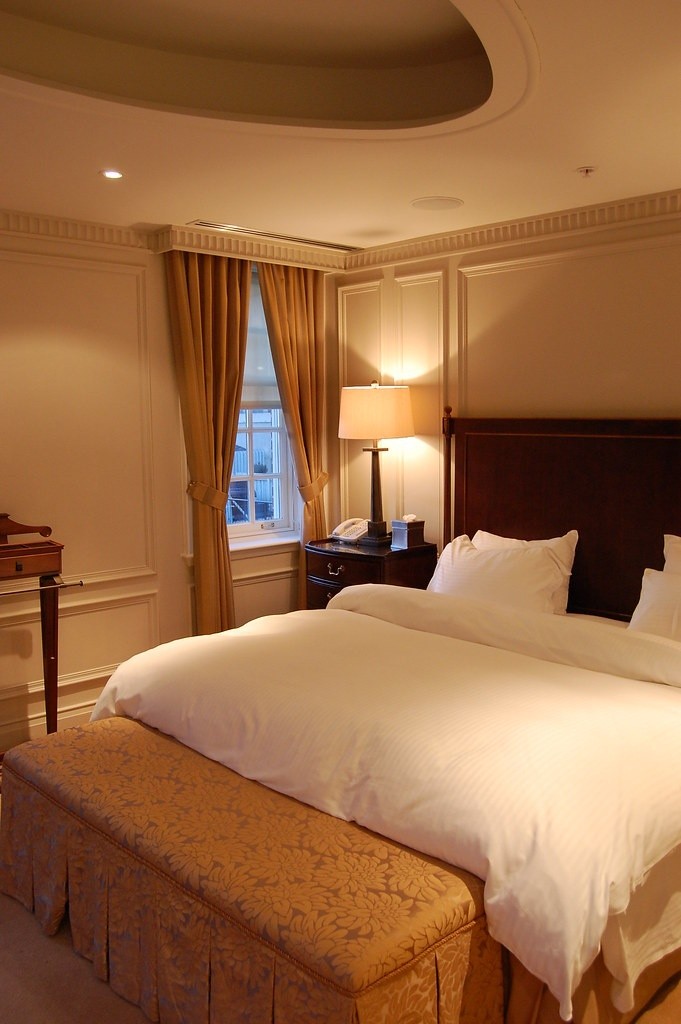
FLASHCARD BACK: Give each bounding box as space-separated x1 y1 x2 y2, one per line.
330 517 371 543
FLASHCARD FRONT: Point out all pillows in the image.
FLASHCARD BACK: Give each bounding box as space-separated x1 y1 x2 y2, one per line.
627 566 681 644
662 533 681 578
426 534 567 616
471 528 579 609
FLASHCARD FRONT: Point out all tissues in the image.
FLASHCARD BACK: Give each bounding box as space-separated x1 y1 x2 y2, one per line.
390 514 426 551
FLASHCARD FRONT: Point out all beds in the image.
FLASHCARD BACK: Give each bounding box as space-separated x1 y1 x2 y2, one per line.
0 407 681 1024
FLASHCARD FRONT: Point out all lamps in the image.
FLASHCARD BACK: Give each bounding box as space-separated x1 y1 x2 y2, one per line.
339 381 420 546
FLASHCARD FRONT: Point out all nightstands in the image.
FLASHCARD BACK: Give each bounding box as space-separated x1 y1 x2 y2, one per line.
303 537 439 614
